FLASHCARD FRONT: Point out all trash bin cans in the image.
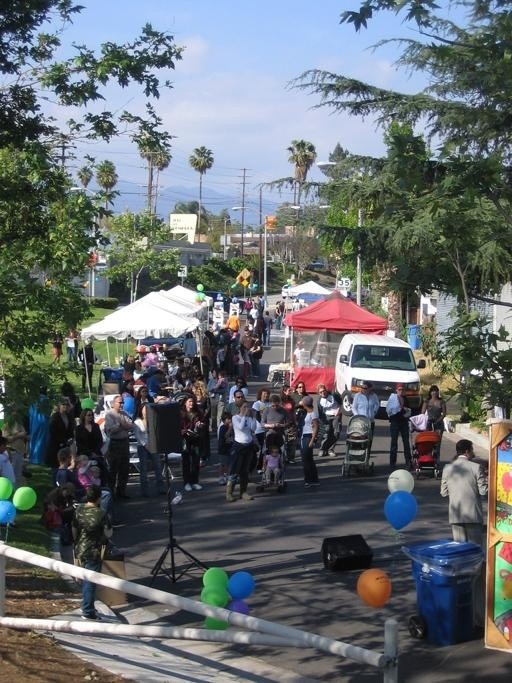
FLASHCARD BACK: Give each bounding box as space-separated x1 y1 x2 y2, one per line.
401 539 484 647
408 325 421 349
102 367 124 383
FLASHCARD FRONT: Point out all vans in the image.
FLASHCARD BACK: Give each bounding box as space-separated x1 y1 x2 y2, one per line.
282 284 296 298
333 332 426 414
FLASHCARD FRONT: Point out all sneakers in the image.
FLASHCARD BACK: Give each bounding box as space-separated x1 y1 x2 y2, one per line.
219 479 225 485
82 614 103 621
328 449 336 456
192 483 202 489
318 449 326 456
304 482 312 488
185 482 192 491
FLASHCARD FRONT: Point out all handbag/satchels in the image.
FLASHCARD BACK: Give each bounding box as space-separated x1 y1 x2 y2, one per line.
47 511 62 527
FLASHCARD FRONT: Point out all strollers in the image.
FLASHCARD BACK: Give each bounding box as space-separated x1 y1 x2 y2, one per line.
254 427 288 491
342 415 378 478
404 417 444 480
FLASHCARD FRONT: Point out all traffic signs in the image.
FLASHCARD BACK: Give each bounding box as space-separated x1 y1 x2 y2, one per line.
338 278 350 286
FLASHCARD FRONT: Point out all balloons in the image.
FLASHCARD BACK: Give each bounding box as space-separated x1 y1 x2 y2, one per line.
357 570 392 611
200 568 255 630
0 478 38 524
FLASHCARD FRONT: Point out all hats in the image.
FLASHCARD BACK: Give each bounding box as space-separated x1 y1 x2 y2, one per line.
61 481 77 496
56 398 70 405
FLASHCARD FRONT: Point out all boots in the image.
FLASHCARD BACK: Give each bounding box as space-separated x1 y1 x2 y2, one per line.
240 479 253 499
226 480 236 501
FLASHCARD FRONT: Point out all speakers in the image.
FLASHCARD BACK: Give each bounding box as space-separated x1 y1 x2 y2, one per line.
146 402 182 454
320 535 371 569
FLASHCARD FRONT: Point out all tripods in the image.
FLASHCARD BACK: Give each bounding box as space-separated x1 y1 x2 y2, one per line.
144 457 210 599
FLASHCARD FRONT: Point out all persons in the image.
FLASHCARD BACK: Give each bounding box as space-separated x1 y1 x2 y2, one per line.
25 278 446 547
71 485 112 621
441 441 489 546
0 436 16 483
2 410 26 476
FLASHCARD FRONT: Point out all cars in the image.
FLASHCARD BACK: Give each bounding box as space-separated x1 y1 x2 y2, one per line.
202 290 246 314
306 260 324 271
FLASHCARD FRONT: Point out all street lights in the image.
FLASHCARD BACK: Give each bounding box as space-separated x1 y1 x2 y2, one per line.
319 205 348 220
232 205 299 302
316 162 363 305
70 186 98 303
223 217 237 260
